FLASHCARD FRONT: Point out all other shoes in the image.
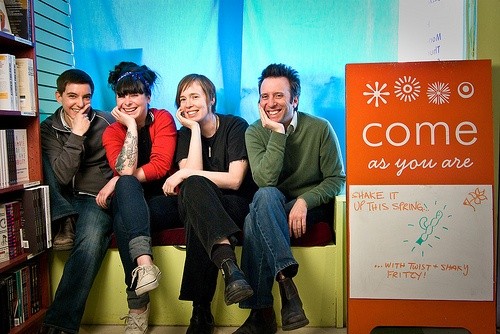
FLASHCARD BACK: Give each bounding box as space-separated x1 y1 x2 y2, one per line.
186 304 215 334
221 257 255 306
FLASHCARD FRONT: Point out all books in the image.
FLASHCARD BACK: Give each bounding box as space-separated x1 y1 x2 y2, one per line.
0 185 52 263
0 0 32 41
0 128 29 188
0 54 36 113
0 263 42 334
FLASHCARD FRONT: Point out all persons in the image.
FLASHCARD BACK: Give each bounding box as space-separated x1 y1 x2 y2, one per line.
40 69 116 334
162 74 253 334
96 62 178 334
232 64 346 334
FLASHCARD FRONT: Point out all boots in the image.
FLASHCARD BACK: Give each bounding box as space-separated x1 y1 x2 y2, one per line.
278 275 310 331
231 304 278 334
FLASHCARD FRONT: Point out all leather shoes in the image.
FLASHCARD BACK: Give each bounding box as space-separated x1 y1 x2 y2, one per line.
53 215 77 251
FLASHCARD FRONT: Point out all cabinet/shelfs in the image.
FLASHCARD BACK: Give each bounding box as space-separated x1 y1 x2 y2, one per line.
0 0 51 334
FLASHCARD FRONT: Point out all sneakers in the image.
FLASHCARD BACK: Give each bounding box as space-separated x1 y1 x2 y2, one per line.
130 264 162 296
120 299 151 334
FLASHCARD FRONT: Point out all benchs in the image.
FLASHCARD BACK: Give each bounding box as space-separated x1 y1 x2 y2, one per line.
48 197 346 328
